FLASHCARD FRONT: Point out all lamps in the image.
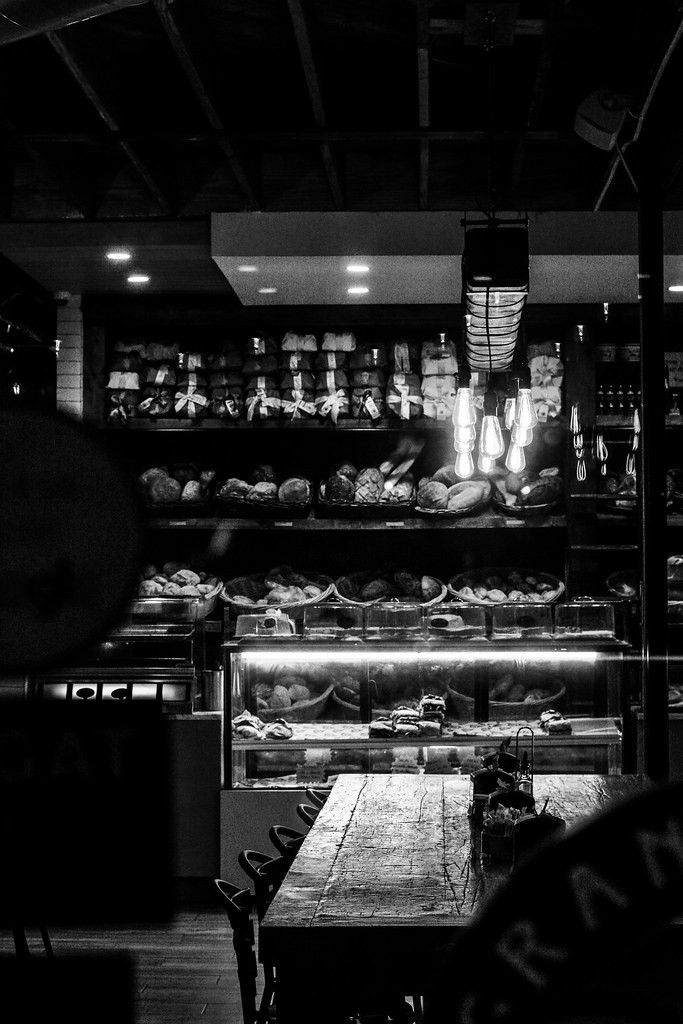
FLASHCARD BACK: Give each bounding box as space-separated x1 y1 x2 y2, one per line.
573 92 626 151
460 217 530 372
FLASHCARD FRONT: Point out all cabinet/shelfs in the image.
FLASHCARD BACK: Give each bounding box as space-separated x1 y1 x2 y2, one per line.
103 418 683 530
218 639 639 899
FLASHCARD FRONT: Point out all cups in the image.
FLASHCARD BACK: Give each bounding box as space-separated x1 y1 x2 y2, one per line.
481 829 513 861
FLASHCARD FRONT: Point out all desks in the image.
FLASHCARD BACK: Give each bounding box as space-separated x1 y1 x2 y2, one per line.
259 774 683 1024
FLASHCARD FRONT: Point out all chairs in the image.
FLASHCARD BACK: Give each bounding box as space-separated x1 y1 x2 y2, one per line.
269 824 306 867
212 878 276 1024
305 788 327 809
295 803 320 829
237 850 285 921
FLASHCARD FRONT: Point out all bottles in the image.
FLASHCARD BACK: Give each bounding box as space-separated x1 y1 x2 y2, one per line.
515 752 532 796
